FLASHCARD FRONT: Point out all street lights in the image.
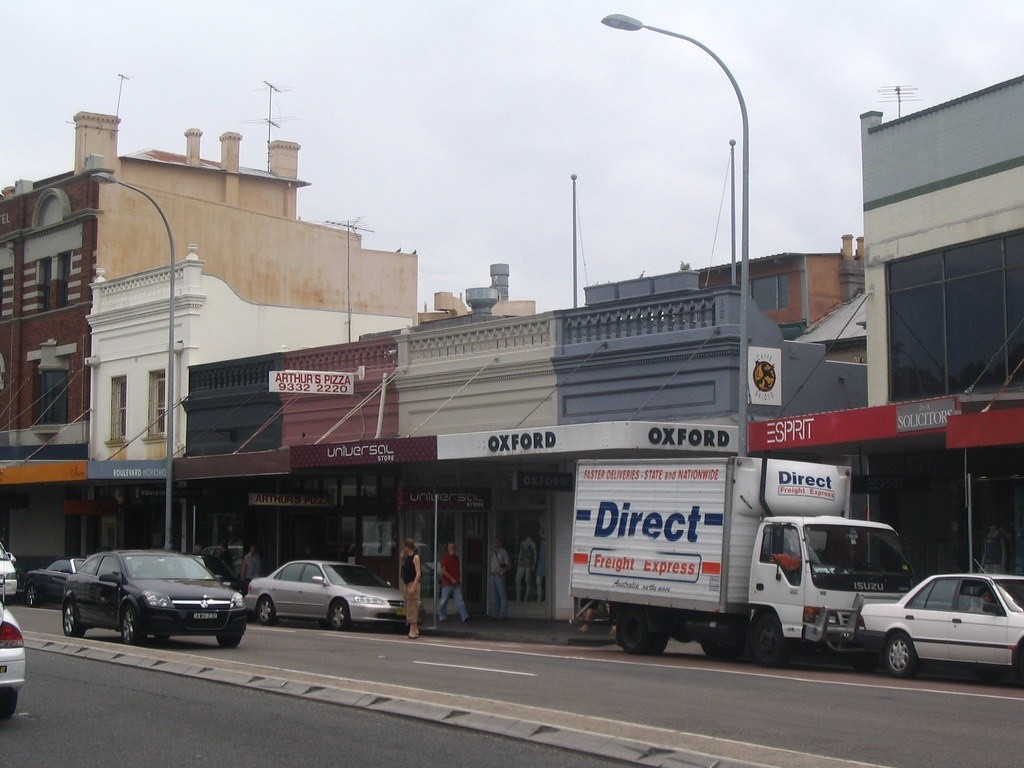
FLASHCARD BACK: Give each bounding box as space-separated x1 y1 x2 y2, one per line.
601 15 752 457
91 171 176 551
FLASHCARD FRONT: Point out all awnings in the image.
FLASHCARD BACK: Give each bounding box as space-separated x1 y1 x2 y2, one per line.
0 422 740 483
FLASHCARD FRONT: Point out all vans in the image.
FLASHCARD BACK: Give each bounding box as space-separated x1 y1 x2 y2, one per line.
0 541 18 598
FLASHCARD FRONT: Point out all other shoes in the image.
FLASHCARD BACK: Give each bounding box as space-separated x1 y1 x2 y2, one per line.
405 634 418 639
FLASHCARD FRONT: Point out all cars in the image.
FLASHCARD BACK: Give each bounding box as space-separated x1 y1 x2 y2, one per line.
0 600 26 720
24 557 89 608
856 572 1024 688
61 549 248 649
244 559 422 632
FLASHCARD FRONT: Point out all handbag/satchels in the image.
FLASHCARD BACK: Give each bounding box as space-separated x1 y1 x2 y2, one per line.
500 563 508 569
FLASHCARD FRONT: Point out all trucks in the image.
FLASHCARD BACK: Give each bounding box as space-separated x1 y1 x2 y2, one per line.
567 456 917 671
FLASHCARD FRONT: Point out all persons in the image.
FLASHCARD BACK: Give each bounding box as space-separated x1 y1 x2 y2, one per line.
487 536 511 621
401 539 421 639
192 541 260 598
578 599 616 635
945 522 1014 574
980 587 997 612
513 535 547 602
438 542 472 623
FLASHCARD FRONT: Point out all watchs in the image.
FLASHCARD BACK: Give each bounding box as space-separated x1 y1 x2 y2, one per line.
501 571 504 575
